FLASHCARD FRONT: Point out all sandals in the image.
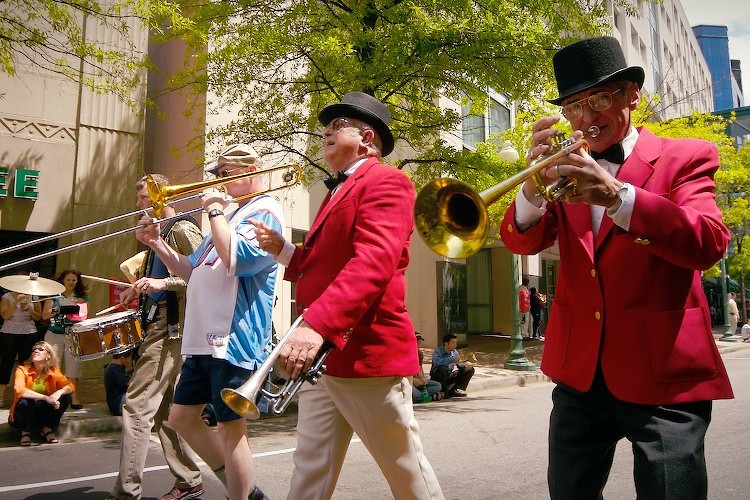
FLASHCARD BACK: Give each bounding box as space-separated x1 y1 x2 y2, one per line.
40 429 58 443
20 433 30 446
428 392 442 400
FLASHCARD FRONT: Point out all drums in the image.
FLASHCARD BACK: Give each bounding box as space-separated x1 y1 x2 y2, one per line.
69 309 144 363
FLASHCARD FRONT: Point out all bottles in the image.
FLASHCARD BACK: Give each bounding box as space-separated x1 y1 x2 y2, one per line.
423 387 428 403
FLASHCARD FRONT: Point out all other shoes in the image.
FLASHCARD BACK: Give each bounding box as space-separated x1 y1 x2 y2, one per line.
70 403 82 409
522 337 534 341
533 337 539 340
157 479 204 500
248 486 264 500
450 390 467 397
539 336 545 339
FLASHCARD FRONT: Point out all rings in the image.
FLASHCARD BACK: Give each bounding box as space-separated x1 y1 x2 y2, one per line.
528 149 537 160
571 184 577 196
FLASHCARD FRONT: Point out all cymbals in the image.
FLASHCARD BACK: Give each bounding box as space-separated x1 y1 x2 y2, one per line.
0 272 67 296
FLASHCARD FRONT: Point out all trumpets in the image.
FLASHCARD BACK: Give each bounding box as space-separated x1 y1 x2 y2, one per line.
414 126 602 260
220 306 334 419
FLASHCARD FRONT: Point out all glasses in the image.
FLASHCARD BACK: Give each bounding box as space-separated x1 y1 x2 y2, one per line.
216 166 245 179
321 118 367 139
33 348 49 354
561 84 633 122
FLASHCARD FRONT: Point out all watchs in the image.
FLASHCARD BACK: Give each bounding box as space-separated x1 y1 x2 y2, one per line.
162 277 170 290
207 208 224 219
605 180 629 215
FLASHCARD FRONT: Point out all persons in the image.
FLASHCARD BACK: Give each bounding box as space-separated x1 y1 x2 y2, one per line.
709 291 750 342
136 143 286 500
247 90 445 500
518 277 547 342
111 173 205 500
104 350 136 416
8 341 75 447
0 269 89 409
411 332 479 404
498 35 735 500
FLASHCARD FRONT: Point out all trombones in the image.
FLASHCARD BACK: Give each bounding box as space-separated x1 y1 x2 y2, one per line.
0 163 301 271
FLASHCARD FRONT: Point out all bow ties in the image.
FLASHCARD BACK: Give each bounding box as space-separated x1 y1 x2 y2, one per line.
589 142 625 164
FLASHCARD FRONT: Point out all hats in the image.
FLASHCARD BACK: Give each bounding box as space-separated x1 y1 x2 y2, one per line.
546 35 645 106
208 143 262 175
414 331 424 340
318 92 395 158
